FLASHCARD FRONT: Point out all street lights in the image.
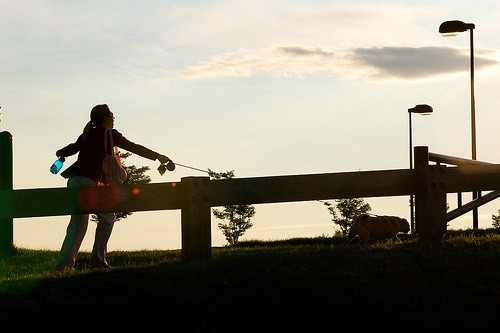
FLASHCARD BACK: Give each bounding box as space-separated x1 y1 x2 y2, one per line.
438 20 478 231
408 104 434 234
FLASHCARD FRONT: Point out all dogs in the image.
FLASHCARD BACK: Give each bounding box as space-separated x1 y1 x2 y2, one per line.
343 213 409 244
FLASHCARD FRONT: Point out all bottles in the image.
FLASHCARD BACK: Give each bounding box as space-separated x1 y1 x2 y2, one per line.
50 157 65 174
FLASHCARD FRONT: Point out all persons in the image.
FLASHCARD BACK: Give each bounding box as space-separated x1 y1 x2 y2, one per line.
55 104 170 271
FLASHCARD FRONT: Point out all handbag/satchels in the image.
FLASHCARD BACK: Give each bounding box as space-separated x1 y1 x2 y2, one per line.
102 128 127 184
